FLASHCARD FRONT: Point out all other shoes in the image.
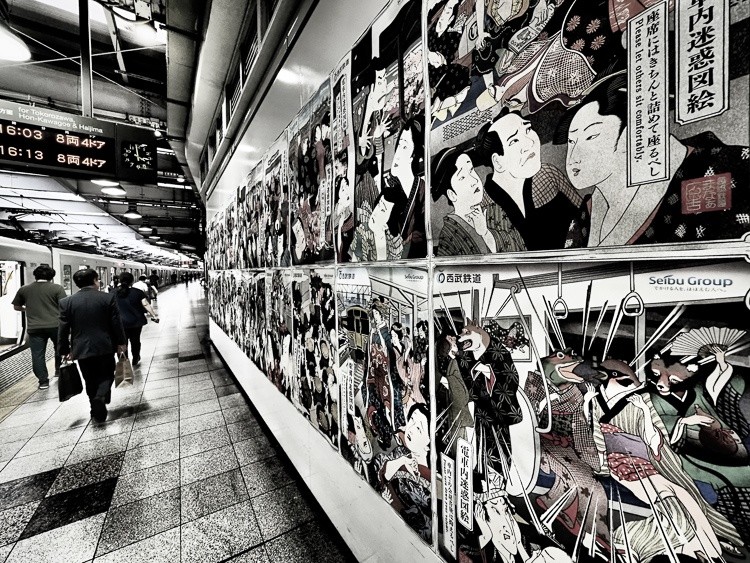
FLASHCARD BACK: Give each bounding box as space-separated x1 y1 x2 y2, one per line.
53 365 60 377
89 398 108 425
132 354 141 365
37 378 49 389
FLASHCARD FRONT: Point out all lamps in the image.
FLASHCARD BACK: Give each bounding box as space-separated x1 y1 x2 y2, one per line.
149 228 160 239
139 219 152 231
124 203 142 219
101 186 126 195
156 239 165 244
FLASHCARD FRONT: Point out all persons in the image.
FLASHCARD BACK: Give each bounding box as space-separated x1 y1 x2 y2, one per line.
11 265 160 423
170 270 204 288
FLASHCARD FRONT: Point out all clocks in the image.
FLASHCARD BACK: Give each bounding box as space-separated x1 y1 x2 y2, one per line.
121 140 155 174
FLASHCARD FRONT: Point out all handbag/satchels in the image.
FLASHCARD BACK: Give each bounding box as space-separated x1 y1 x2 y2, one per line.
114 351 134 389
58 355 83 402
